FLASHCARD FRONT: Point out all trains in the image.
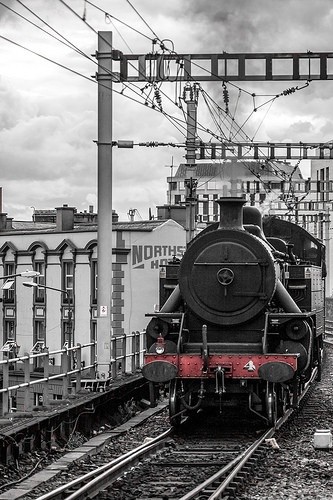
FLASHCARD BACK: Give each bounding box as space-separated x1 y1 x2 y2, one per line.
141 197 328 429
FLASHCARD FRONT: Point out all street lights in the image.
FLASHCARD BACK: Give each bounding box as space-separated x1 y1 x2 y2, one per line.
22 283 72 394
